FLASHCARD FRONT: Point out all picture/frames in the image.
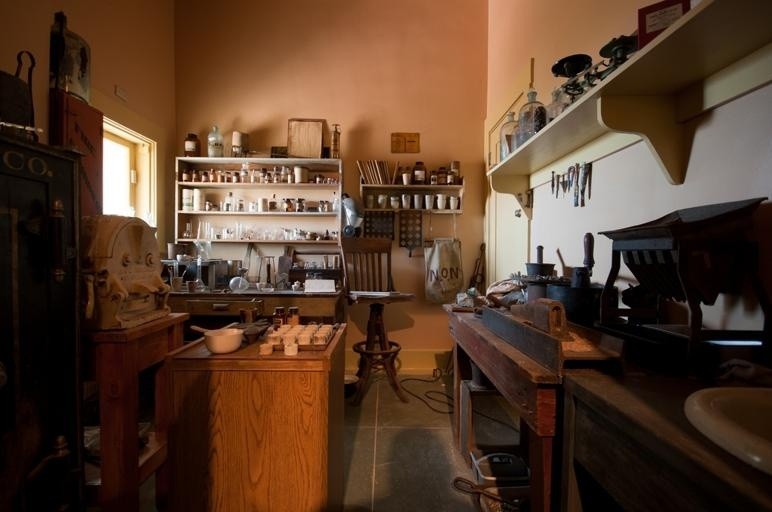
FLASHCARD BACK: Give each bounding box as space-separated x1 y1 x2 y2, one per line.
287 118 325 158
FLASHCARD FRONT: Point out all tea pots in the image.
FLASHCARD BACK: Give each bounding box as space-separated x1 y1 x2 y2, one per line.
258 255 278 288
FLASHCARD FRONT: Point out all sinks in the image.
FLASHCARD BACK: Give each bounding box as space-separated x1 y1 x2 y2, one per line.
683 386 772 474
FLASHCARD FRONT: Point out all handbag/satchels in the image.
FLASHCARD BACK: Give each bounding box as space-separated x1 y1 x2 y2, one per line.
423 240 464 304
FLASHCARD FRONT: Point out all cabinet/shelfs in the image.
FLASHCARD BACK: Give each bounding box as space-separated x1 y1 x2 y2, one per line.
0 129 85 511
562 374 772 511
174 157 344 287
359 175 465 214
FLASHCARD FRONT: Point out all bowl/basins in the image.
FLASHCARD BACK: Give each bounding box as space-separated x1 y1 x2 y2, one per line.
167 242 189 262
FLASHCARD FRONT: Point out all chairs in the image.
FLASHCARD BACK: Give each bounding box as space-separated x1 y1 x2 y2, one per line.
341 238 416 406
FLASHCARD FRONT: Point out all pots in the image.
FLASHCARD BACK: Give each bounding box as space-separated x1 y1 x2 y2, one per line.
189 324 245 354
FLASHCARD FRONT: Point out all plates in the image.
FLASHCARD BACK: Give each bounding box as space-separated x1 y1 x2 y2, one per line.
261 288 274 293
344 374 360 385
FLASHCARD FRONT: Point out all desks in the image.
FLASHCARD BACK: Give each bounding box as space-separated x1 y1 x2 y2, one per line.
155 323 347 512
167 288 344 324
444 304 645 511
85 312 190 511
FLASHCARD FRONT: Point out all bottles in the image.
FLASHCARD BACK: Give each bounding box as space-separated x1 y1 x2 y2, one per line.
182 222 336 240
367 192 460 211
304 254 340 270
183 132 198 156
207 126 223 157
499 88 563 162
397 160 455 185
271 306 300 330
177 254 195 263
206 191 338 213
182 165 338 185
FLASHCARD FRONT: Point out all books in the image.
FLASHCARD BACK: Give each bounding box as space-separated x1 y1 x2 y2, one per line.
356 160 400 185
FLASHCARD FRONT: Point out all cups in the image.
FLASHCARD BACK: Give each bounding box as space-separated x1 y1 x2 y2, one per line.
294 282 300 289
258 324 334 358
292 285 297 292
239 307 257 324
256 282 265 290
171 276 183 292
186 280 196 292
